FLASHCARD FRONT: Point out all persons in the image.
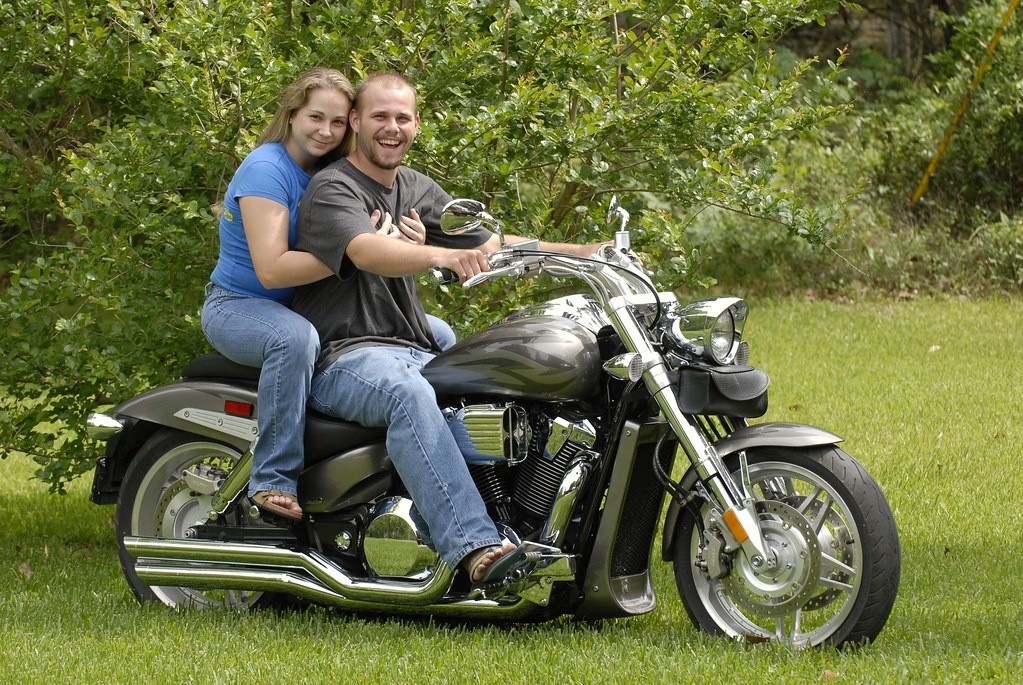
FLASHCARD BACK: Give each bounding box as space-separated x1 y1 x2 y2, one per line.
202 67 425 515
292 72 645 590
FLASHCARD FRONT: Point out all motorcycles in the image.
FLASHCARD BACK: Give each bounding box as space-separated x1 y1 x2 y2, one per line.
87 194 904 658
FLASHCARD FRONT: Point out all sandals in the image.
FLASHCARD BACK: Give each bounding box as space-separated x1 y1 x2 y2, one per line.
466 540 528 585
251 490 304 520
